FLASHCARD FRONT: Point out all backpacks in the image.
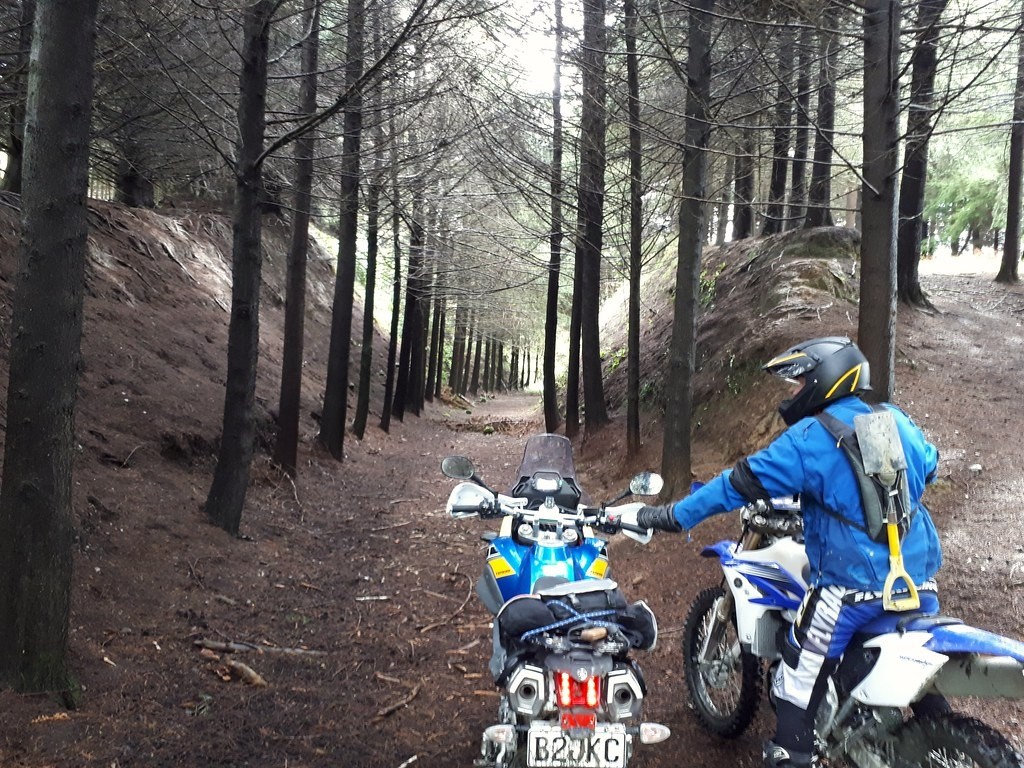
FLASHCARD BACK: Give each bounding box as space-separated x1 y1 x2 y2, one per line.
802 402 918 546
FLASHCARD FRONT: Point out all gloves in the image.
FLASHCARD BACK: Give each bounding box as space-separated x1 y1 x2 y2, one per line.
636 502 683 534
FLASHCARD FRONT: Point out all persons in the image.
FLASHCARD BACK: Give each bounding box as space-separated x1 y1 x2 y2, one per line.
620 336 942 768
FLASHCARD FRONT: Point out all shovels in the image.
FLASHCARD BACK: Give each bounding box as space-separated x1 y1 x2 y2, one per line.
853 409 921 613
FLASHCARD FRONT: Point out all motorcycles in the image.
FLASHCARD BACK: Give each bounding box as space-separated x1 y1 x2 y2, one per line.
439 433 672 768
681 472 1024 768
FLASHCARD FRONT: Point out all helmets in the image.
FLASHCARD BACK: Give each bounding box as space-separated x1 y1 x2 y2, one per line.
761 335 874 427
